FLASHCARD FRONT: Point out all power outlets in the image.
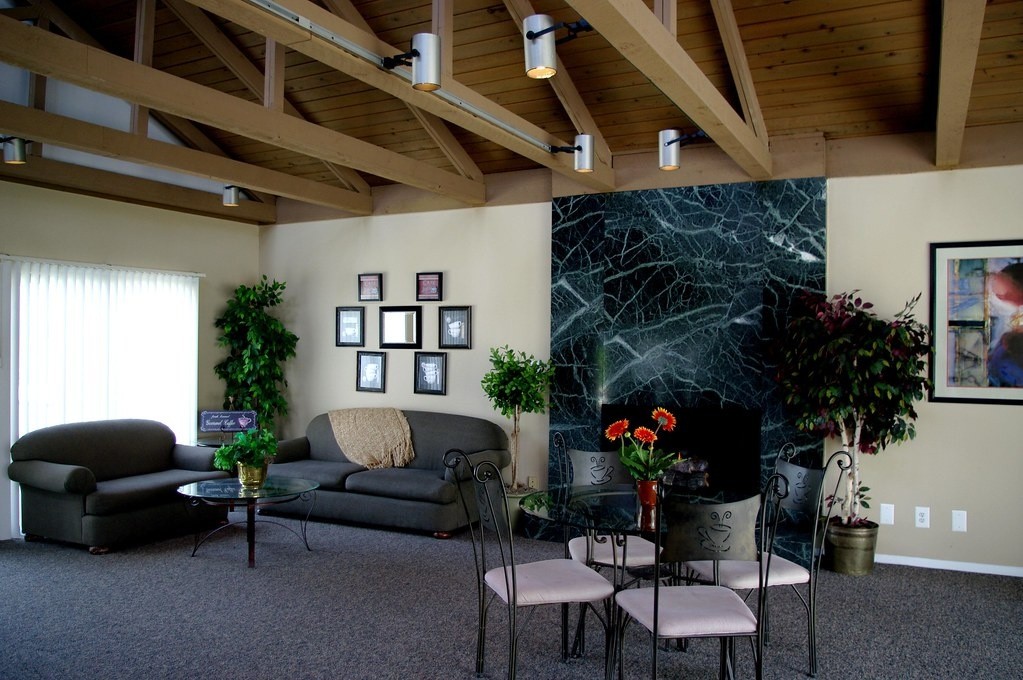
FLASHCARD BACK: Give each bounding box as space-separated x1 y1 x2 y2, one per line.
915 506 930 528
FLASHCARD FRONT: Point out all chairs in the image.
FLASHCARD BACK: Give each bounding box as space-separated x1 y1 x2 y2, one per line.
614 470 789 680
552 430 685 656
678 442 853 680
442 448 617 680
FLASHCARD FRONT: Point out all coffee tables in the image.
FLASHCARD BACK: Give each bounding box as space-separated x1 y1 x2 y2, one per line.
176 477 319 569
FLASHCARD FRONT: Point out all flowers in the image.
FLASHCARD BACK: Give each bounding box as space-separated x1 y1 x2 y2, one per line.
604 407 687 482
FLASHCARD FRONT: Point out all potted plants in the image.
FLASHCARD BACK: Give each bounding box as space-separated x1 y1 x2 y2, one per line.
779 286 935 574
480 345 557 530
215 424 279 490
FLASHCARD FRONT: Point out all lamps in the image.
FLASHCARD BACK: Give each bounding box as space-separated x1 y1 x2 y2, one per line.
384 33 442 92
0 133 32 165
659 128 712 170
222 182 240 206
549 133 594 172
522 13 593 78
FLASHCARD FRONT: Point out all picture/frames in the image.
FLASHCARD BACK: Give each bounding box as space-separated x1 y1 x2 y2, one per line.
335 306 365 347
413 351 447 395
356 351 386 392
439 307 472 350
357 273 383 301
415 272 444 301
928 239 1023 406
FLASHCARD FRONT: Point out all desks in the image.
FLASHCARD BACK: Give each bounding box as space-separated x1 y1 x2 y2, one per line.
195 438 237 448
519 482 787 680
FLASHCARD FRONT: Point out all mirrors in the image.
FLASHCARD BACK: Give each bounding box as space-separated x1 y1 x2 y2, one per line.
379 306 422 349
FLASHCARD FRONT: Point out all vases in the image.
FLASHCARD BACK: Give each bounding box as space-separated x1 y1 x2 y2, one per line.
636 480 658 506
638 505 656 530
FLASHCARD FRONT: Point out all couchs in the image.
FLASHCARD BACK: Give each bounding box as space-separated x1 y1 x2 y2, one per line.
7 419 227 553
257 409 512 539
